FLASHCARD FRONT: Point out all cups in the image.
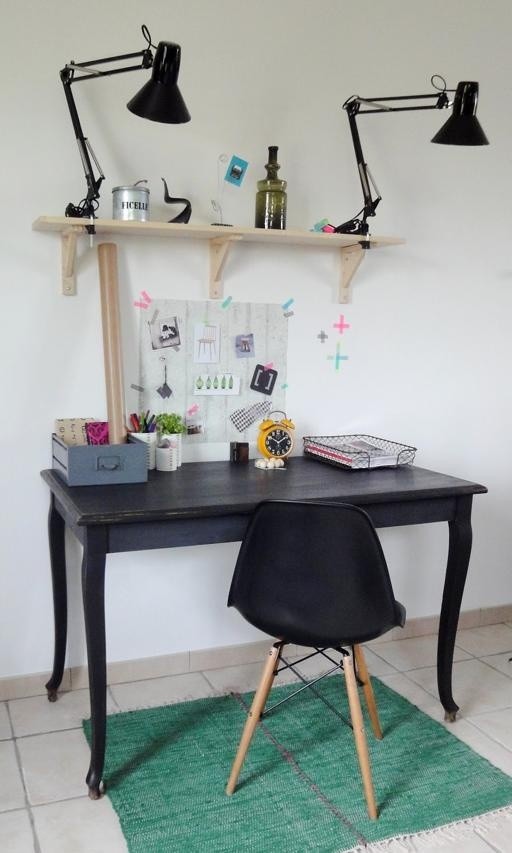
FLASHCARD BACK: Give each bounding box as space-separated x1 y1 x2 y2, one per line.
229 441 249 465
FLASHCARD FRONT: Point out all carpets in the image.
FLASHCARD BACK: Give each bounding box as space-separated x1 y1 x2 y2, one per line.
83 673 512 853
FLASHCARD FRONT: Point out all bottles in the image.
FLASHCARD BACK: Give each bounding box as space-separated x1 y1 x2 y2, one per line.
255 146 288 230
112 180 150 222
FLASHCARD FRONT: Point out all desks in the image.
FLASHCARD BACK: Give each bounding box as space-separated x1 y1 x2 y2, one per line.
41 456 488 801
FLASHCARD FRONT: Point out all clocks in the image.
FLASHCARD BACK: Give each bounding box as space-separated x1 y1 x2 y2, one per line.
258 409 295 460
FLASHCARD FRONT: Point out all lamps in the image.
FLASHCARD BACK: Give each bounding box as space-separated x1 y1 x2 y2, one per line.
60 24 192 218
334 74 490 234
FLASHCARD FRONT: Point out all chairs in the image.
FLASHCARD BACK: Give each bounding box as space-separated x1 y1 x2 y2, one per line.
225 500 406 819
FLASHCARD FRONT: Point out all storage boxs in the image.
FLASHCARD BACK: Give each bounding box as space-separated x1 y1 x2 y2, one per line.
52 432 149 487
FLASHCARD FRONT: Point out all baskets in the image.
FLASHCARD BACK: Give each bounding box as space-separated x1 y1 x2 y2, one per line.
303 435 417 469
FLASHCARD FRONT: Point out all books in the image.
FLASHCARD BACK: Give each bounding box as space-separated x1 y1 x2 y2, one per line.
305 439 403 469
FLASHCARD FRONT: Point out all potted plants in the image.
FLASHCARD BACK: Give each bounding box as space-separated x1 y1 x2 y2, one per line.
156 413 186 467
